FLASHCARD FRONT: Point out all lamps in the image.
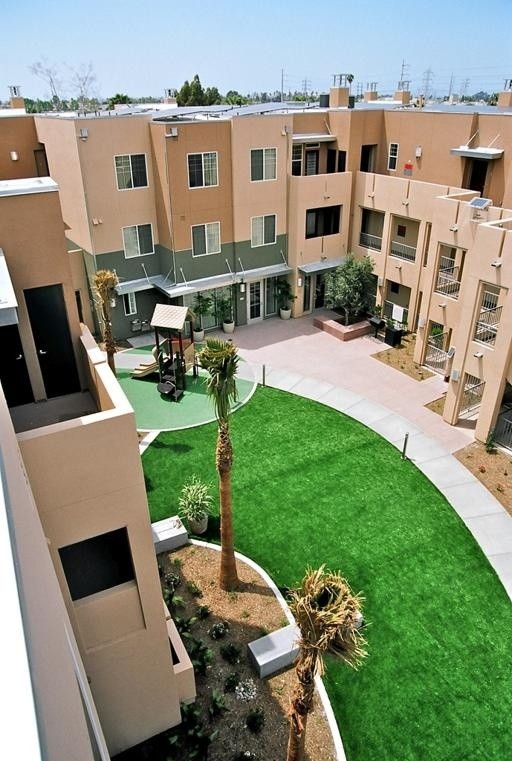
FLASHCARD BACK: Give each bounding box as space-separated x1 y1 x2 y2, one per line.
110 299 116 307
378 278 384 287
240 284 246 293
419 318 425 327
298 277 303 287
450 369 460 381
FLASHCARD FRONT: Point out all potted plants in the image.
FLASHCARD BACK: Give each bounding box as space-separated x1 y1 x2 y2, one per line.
274 280 297 320
190 292 214 342
177 472 219 535
210 287 235 334
